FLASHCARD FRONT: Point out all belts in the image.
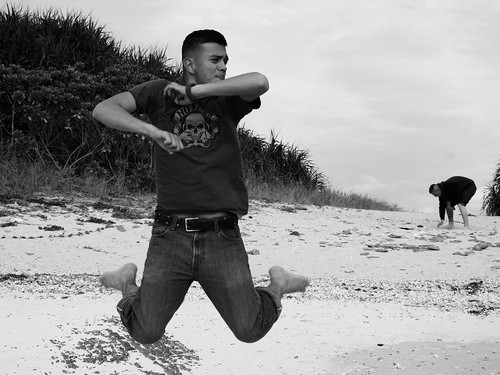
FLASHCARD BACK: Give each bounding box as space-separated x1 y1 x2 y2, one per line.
154 213 239 231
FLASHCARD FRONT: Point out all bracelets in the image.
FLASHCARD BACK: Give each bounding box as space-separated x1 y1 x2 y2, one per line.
185 83 203 104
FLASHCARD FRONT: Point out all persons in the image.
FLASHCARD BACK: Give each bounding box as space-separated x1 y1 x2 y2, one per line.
90 29 311 346
429 176 478 229
